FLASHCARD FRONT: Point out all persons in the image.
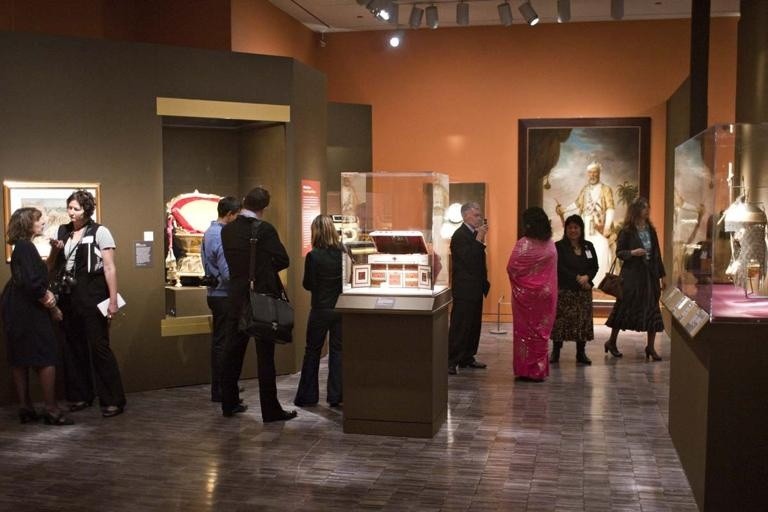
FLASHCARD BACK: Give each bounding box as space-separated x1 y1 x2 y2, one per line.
602 195 668 363
218 186 298 426
293 213 347 410
562 161 622 276
0 206 78 425
200 196 247 402
448 200 489 376
505 206 558 382
551 214 599 364
49 190 127 418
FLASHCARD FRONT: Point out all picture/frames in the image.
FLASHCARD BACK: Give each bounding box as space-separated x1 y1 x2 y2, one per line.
2 179 101 266
515 115 652 310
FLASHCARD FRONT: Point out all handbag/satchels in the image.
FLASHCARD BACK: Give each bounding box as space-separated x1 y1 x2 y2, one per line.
598 253 627 299
238 288 294 345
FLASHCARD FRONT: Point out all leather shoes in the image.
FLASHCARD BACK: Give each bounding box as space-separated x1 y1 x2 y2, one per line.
576 352 593 365
71 397 93 410
102 402 125 417
547 352 561 362
214 383 249 415
448 364 456 374
459 358 487 369
260 407 297 422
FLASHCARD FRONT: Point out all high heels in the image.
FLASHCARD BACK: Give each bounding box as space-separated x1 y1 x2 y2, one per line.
645 346 662 361
18 406 41 423
42 409 74 426
604 340 623 357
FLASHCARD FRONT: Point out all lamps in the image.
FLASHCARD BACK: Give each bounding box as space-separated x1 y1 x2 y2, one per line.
362 1 628 49
